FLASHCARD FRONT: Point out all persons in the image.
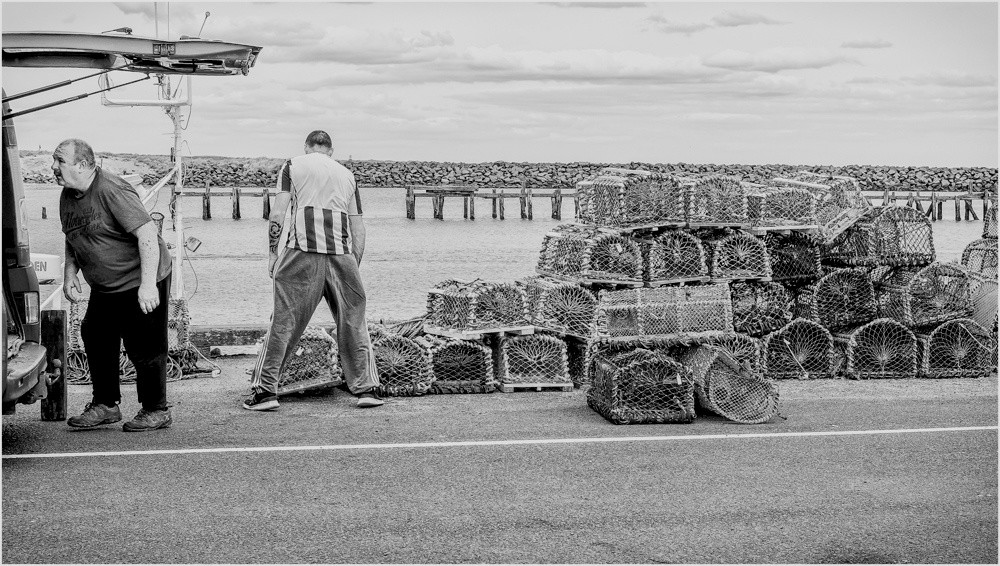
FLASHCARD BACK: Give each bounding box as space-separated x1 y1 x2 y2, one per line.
243 130 385 409
52 140 172 432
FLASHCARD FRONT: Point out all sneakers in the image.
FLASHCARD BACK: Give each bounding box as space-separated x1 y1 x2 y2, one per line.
123 408 172 432
356 393 384 408
243 392 280 410
67 402 122 427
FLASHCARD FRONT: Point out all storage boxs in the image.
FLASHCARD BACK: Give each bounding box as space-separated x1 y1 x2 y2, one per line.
68 168 998 423
